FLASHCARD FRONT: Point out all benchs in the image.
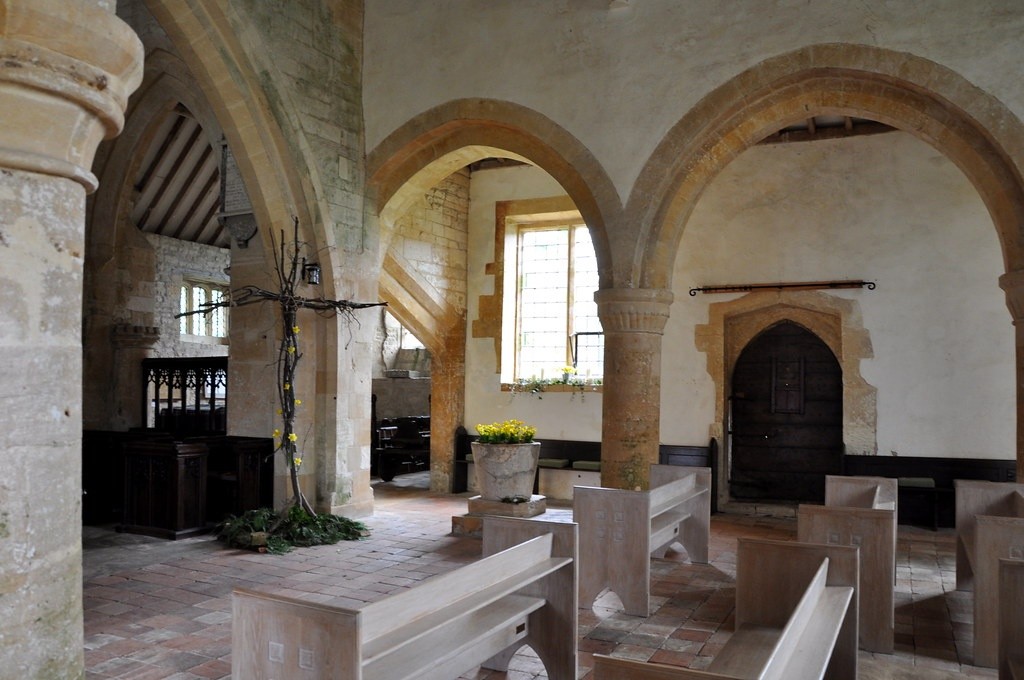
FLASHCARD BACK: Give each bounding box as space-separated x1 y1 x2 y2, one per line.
374 447 431 476
376 425 398 447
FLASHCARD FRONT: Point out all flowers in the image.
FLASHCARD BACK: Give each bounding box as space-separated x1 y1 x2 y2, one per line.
561 365 575 373
473 420 537 444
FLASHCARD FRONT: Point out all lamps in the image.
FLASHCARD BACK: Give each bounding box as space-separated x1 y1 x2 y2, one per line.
302 257 321 284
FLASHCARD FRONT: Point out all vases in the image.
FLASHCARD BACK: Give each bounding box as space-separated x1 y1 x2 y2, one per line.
562 374 570 384
471 442 542 502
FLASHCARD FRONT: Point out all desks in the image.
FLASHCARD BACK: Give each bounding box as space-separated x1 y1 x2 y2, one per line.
82 403 431 542
573 464 712 618
998 557 1024 680
231 515 578 680
592 536 860 680
797 475 897 655
956 480 1024 668
